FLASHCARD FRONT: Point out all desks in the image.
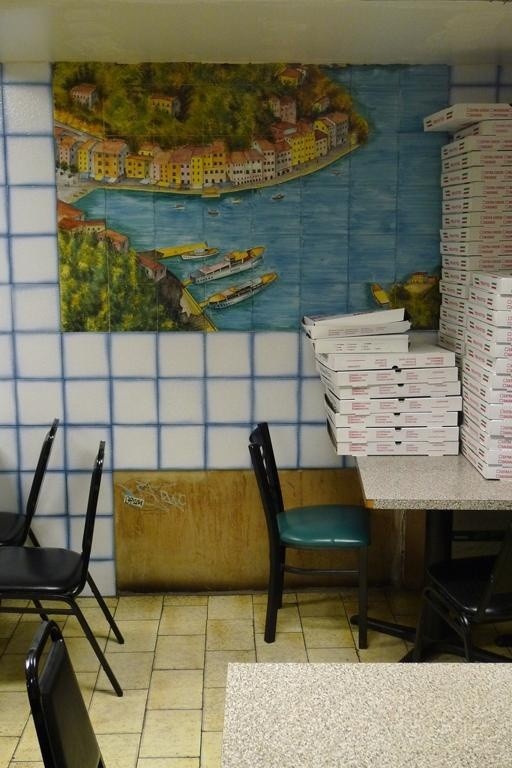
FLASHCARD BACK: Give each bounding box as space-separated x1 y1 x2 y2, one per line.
348 454 512 662
222 660 510 766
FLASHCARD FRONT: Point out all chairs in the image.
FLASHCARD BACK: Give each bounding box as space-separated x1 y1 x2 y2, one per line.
416 533 512 663
23 615 109 767
248 420 370 650
0 417 59 623
1 441 125 697
